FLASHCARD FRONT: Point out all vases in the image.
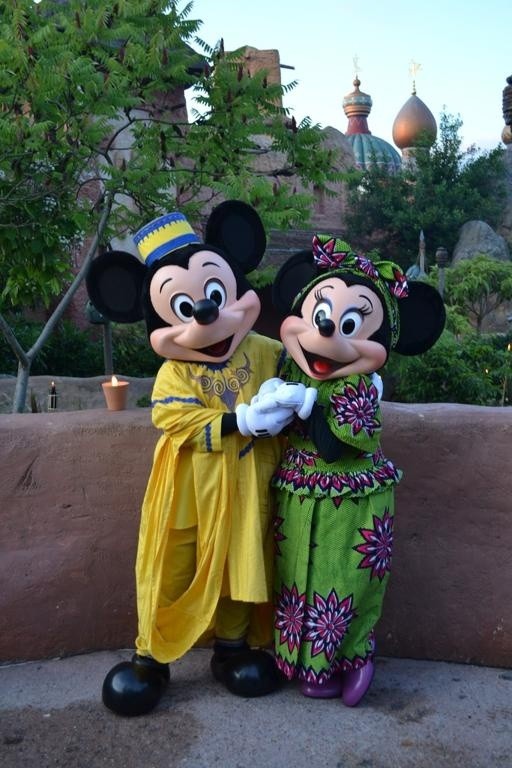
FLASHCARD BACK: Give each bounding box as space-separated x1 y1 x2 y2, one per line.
100 381 130 412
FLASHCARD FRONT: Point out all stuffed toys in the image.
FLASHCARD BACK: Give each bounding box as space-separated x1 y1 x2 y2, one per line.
256 232 445 708
86 194 390 715
